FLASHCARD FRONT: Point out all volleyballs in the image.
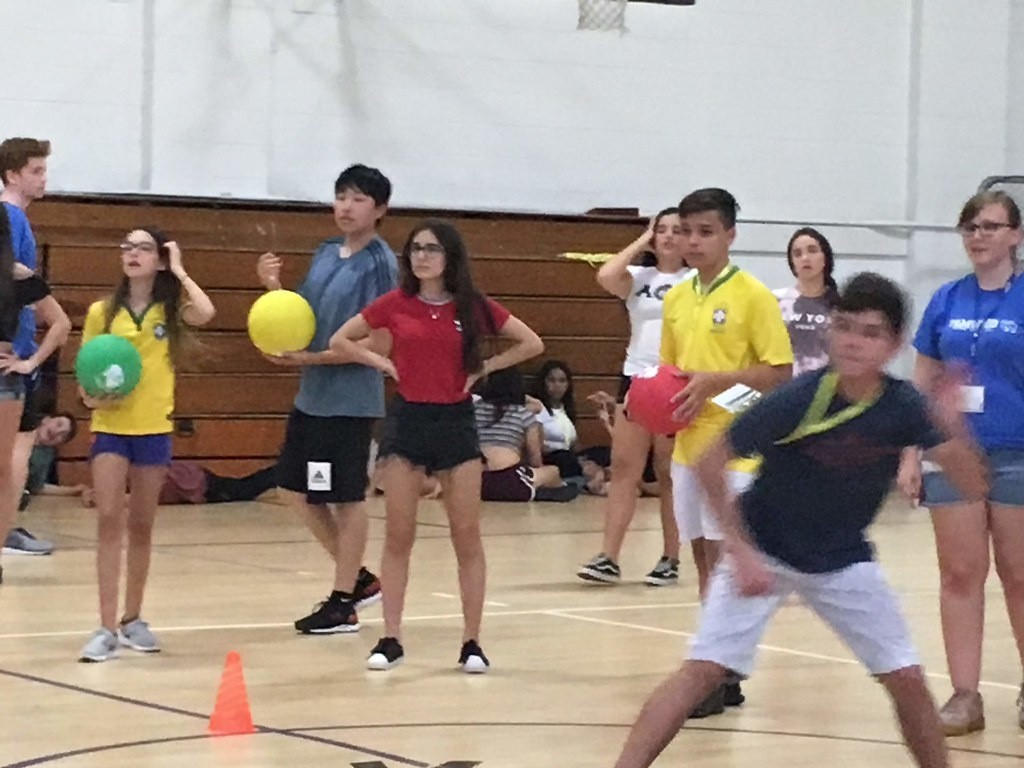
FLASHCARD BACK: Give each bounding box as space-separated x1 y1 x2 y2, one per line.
74 333 143 400
247 289 316 357
627 362 694 435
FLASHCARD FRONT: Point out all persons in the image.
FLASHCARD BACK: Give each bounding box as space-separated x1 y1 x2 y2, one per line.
576 207 691 584
771 226 842 377
18 411 78 510
80 462 283 508
1 138 56 556
473 367 561 502
895 190 1024 733
256 163 400 636
0 204 71 585
613 275 987 768
581 367 659 496
329 218 546 673
622 186 792 721
534 359 581 478
77 227 216 662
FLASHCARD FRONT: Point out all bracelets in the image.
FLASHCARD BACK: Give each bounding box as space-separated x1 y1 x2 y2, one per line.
180 273 189 281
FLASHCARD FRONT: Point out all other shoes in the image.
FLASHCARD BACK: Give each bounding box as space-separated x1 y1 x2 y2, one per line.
535 486 579 501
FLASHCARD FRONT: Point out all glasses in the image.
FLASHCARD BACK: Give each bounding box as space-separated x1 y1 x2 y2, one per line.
408 242 446 259
955 222 1014 237
118 241 159 254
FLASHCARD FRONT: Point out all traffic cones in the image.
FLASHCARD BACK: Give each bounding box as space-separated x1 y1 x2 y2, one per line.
206 649 258 734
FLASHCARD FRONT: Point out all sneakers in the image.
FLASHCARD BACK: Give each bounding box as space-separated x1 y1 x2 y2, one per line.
724 683 745 706
644 556 679 585
0 528 53 555
118 620 160 651
689 683 725 719
1016 681 1024 728
935 691 990 738
354 571 383 612
367 637 404 669
458 639 490 673
78 629 120 662
577 552 621 583
295 596 360 633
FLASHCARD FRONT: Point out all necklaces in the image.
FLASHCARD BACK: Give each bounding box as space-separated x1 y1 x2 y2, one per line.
421 288 447 319
